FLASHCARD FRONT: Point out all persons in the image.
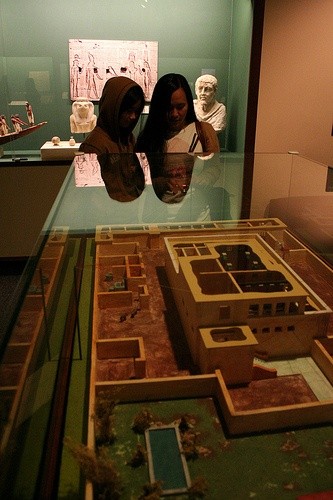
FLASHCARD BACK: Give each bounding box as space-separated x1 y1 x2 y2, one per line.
146 153 196 205
76 76 145 225
192 75 227 134
95 152 145 203
135 74 220 223
